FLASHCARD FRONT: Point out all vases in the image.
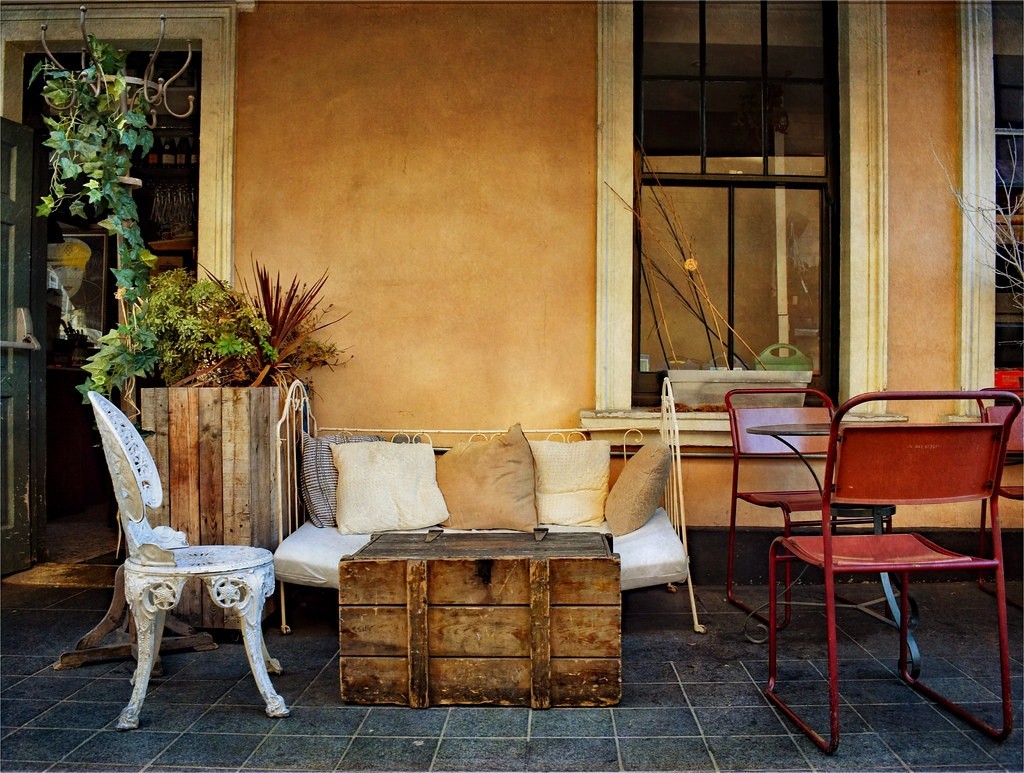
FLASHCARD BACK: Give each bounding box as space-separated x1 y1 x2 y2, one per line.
668 369 813 405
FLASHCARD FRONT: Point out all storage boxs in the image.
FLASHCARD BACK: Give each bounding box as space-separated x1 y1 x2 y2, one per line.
337 527 623 710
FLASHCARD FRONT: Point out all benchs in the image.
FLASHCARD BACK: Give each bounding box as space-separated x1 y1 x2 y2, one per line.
272 379 707 633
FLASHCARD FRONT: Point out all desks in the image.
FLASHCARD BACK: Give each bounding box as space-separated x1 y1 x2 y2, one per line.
745 420 923 681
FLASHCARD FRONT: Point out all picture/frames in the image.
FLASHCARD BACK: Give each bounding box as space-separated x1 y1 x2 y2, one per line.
48 227 108 349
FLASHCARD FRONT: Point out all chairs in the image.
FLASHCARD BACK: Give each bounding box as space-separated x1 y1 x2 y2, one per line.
725 386 893 631
85 389 290 732
975 387 1024 611
764 390 1023 755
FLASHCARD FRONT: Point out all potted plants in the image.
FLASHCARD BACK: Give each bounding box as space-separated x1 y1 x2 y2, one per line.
27 31 162 405
129 263 350 624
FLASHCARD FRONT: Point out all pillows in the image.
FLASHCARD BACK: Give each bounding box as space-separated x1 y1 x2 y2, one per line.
604 439 671 536
437 422 540 534
302 429 387 528
328 441 449 536
528 439 612 526
